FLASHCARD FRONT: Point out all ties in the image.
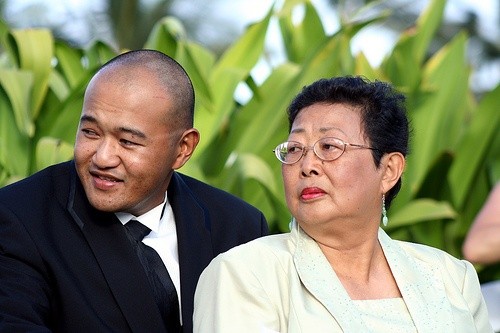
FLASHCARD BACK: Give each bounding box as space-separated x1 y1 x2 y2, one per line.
124 200 182 333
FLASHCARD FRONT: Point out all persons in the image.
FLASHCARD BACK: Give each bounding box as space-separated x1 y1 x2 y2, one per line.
463 183 500 263
0 49 269 333
193 76 494 333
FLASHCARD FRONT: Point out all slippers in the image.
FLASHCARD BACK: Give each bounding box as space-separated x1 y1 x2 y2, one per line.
272 136 383 165
